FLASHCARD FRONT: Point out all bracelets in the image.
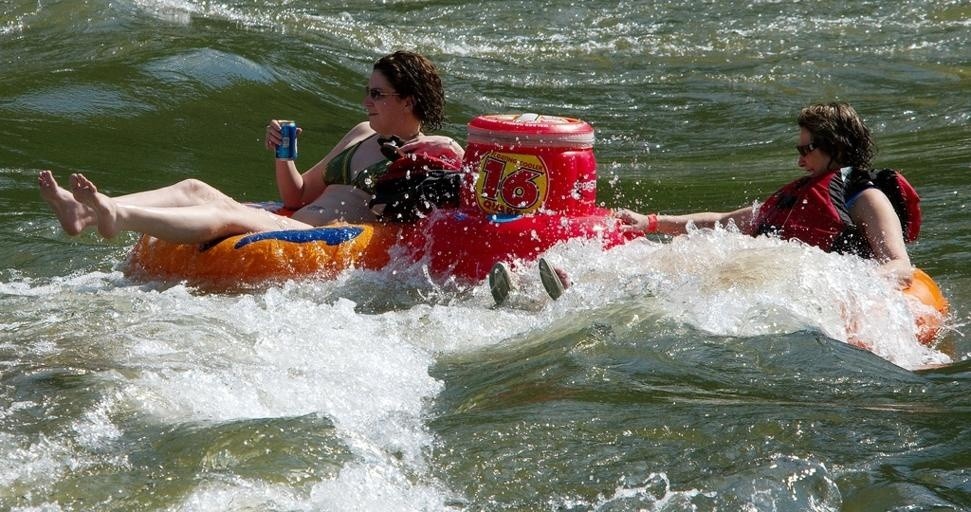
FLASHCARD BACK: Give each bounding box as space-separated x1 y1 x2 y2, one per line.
645 213 658 233
448 140 455 145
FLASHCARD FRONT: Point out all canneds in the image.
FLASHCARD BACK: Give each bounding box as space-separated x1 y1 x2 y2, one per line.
276 119 297 160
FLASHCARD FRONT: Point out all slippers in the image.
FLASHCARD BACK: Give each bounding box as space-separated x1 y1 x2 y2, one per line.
489 262 517 303
540 258 573 300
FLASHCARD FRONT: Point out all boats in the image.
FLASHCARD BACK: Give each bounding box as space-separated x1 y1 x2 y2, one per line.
126 201 951 355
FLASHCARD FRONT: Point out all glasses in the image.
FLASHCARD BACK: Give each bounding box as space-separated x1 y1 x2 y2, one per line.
797 142 817 157
366 86 400 100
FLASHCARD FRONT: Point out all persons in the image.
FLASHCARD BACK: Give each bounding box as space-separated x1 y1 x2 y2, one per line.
37 49 465 245
489 100 913 305
